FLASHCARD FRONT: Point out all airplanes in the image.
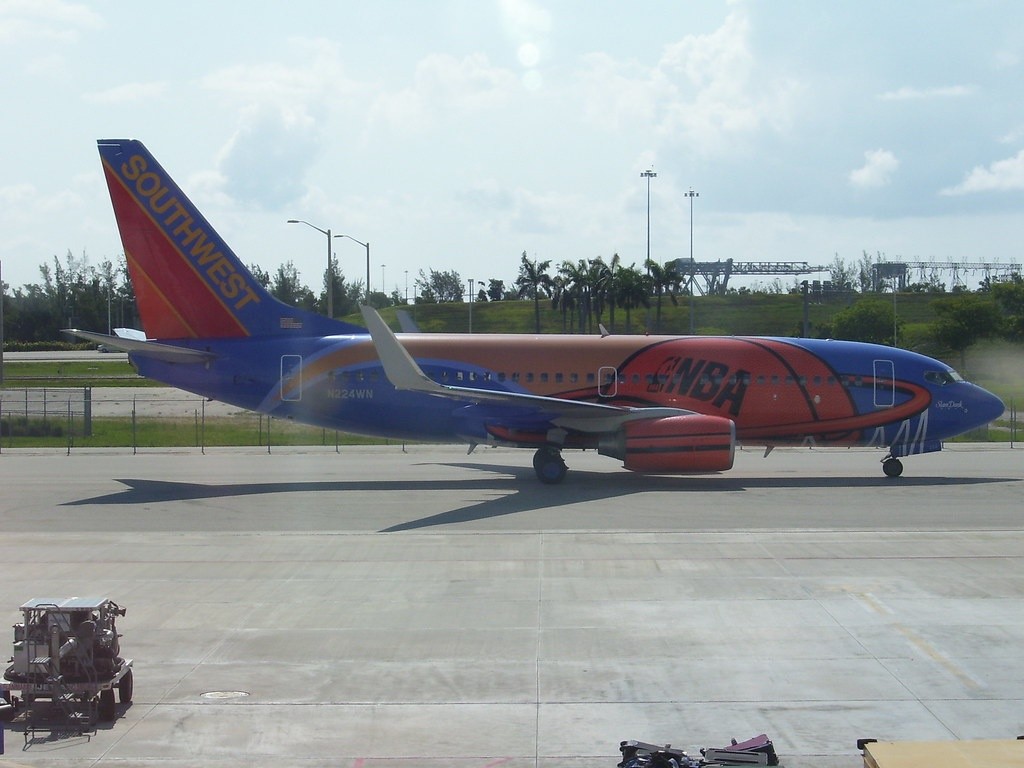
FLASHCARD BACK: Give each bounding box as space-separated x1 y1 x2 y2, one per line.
57 138 1006 486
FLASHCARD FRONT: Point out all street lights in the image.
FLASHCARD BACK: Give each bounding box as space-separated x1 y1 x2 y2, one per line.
684 189 699 335
640 168 657 332
287 219 332 319
335 234 370 307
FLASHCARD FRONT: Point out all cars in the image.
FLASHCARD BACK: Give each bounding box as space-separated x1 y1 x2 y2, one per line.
97 344 125 353
